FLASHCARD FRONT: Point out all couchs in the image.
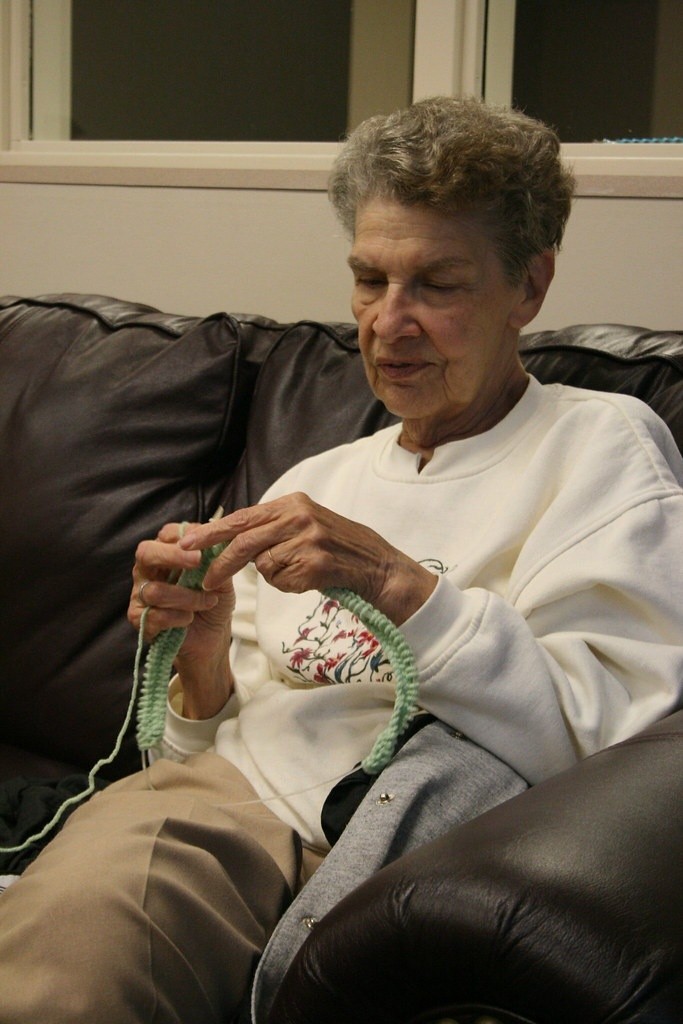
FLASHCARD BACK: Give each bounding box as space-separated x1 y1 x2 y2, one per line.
0 291 683 1024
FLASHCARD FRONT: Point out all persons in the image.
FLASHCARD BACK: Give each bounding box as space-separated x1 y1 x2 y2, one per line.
2 95 683 1024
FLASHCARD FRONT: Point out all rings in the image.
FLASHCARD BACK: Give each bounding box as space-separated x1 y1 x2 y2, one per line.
267 546 286 570
138 580 153 606
